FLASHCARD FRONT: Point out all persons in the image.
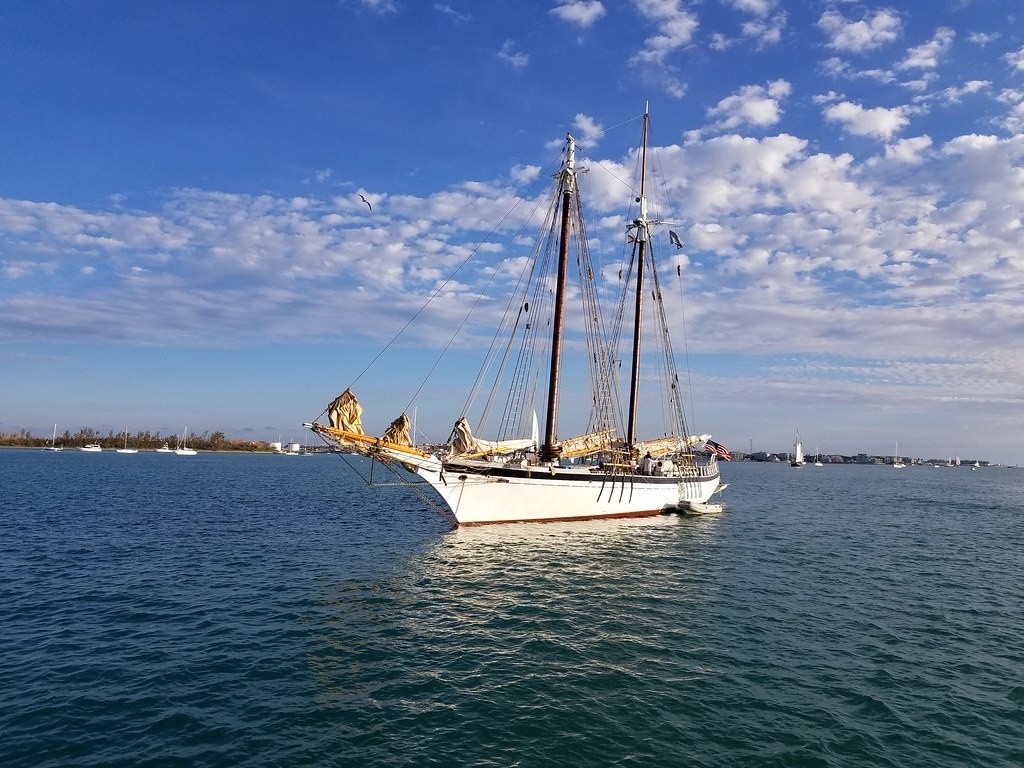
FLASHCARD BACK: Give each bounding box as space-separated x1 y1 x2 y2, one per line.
644 451 651 459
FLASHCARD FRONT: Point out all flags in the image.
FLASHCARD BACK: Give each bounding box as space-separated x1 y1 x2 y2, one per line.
702 439 732 462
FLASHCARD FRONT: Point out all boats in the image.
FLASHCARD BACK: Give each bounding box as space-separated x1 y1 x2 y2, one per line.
676 498 723 516
156 442 173 453
912 464 918 467
900 463 906 467
788 429 806 467
77 442 102 452
974 460 981 467
934 465 941 467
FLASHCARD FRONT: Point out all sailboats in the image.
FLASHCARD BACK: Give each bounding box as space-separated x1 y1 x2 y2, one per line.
945 456 954 466
173 434 184 452
272 433 285 454
891 441 903 468
284 435 298 455
302 434 312 455
174 425 197 455
814 443 823 466
116 426 137 454
44 424 64 452
302 100 722 527
